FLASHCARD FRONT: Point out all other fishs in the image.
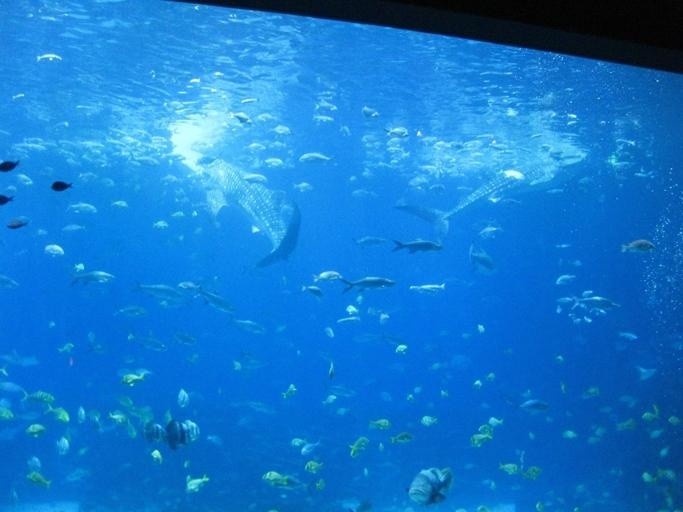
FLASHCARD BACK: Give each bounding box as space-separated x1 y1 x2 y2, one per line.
0 72 682 512
